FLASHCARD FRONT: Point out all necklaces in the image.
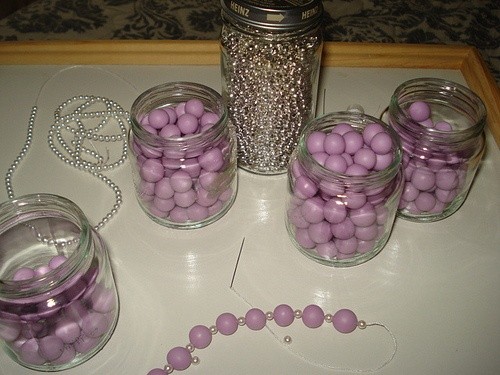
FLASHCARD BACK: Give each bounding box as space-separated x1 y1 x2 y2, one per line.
147 304 367 375
5 95 133 246
